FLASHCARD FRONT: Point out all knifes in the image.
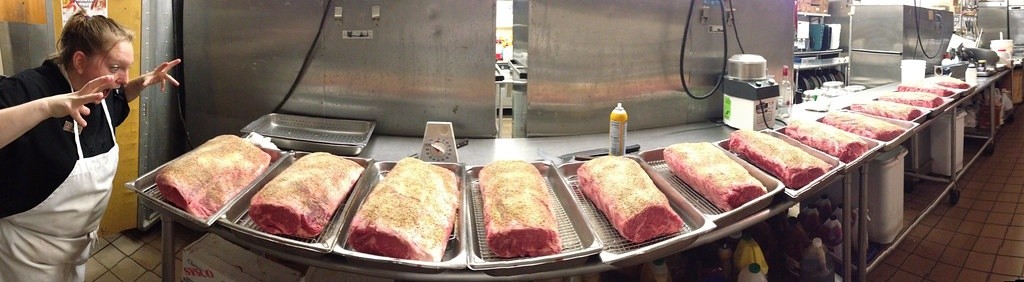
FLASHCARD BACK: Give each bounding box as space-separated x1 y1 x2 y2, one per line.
559 144 640 159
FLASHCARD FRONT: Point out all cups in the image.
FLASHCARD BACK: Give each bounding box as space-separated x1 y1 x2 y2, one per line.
810 22 841 50
901 59 926 84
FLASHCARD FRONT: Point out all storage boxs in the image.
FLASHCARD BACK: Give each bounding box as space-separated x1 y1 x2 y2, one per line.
179 232 316 282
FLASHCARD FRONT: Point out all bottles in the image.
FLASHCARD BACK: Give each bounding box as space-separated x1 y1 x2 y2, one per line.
965 63 977 85
570 193 869 282
609 103 628 155
778 65 791 118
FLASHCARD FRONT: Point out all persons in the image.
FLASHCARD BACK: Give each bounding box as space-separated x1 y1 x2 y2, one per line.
0 11 181 282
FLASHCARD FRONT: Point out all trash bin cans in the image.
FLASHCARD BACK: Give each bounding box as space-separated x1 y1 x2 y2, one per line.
931 110 967 177
799 145 908 244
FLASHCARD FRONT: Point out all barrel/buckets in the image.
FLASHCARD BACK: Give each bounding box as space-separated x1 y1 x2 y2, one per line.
990 40 1013 63
